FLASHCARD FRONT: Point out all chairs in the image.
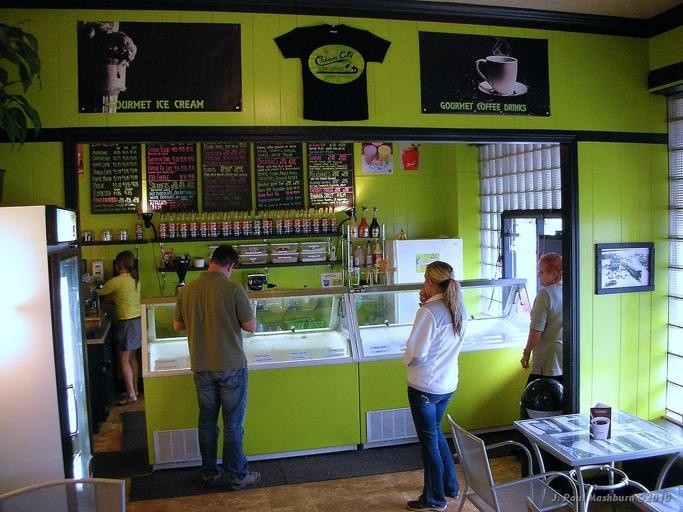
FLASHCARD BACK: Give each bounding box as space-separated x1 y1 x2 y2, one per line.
446 413 579 512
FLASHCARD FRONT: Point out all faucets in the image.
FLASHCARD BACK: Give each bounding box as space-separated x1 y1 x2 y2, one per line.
84 284 104 311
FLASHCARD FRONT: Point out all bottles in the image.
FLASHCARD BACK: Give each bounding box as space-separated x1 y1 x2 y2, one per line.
134 213 143 241
80 230 94 242
100 230 115 241
348 240 381 269
116 229 128 240
157 205 337 240
349 206 380 238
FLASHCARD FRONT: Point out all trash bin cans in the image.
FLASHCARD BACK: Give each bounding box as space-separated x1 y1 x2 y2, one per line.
518 378 565 494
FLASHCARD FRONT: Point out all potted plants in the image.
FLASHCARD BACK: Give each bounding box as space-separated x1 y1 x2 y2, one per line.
1 18 41 204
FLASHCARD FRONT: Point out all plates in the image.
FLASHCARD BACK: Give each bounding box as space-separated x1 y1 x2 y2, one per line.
476 80 528 98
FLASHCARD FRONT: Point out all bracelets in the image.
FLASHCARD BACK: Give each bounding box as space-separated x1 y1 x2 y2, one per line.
523 349 530 356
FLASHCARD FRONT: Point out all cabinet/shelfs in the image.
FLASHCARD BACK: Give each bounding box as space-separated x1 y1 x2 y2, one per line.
347 225 385 284
151 232 344 286
85 322 111 376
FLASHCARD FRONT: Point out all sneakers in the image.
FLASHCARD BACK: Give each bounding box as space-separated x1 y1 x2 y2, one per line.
229 471 262 491
407 494 448 512
200 465 225 488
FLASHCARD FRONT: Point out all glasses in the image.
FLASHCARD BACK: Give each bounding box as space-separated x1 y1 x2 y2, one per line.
539 269 547 273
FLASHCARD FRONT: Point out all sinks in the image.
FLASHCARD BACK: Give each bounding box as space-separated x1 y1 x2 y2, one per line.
84 318 111 345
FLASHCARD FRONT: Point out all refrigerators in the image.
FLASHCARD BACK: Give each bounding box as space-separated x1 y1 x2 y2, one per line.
385 239 465 324
1 205 97 511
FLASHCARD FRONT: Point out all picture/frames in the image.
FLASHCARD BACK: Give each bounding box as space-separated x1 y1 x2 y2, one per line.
595 242 654 294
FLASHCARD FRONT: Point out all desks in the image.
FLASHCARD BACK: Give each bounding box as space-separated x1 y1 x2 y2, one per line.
513 402 682 512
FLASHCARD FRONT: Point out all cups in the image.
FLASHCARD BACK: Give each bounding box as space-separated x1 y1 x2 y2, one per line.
189 257 204 268
589 417 610 440
363 142 391 165
474 54 518 95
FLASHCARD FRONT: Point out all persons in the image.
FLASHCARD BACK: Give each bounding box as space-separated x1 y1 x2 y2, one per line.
173 245 262 491
519 253 563 388
95 251 141 406
403 261 467 511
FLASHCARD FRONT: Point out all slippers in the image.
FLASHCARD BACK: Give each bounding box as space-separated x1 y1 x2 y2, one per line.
120 391 139 396
119 395 137 404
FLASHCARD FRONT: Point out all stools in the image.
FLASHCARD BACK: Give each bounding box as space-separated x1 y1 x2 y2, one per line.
633 483 682 512
